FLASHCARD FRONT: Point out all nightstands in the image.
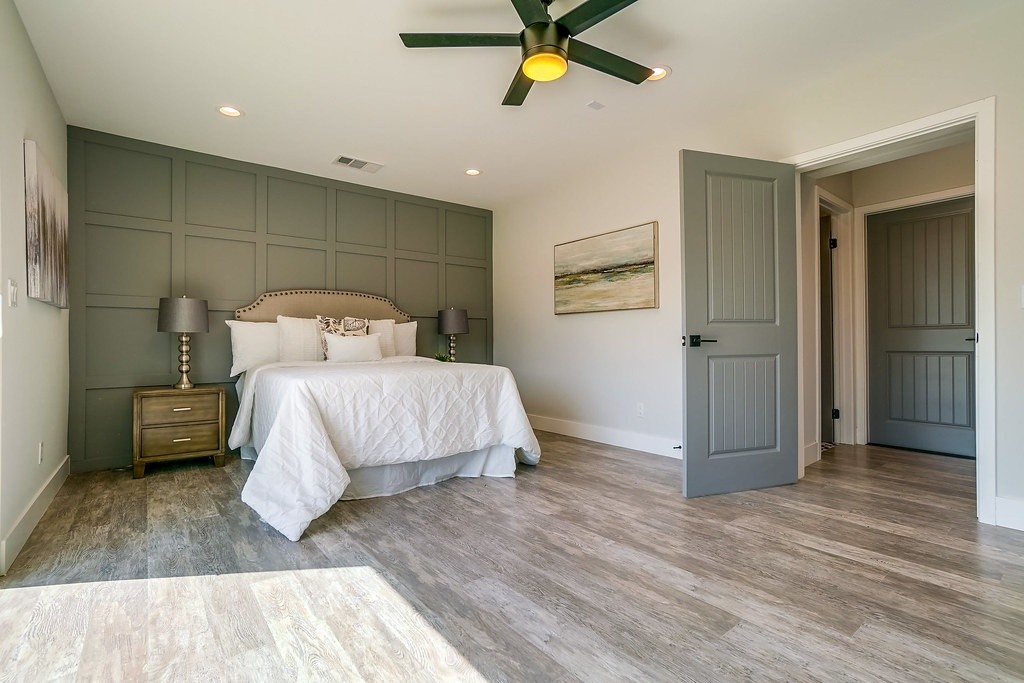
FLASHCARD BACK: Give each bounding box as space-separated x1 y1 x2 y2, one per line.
132 384 224 479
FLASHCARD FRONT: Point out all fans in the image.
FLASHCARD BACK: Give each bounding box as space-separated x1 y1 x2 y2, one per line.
399 0 654 108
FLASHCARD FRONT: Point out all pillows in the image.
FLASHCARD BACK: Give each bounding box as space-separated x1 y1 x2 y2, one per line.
393 319 418 357
323 330 381 364
224 319 279 378
367 318 397 359
316 314 368 363
277 314 325 361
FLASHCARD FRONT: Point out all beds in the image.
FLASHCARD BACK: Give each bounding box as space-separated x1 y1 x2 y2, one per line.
232 289 542 542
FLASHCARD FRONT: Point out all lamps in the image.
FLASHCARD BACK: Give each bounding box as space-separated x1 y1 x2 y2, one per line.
156 293 209 389
437 306 469 362
522 46 568 81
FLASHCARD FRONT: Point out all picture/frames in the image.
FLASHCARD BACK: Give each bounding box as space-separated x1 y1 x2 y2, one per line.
553 220 659 314
